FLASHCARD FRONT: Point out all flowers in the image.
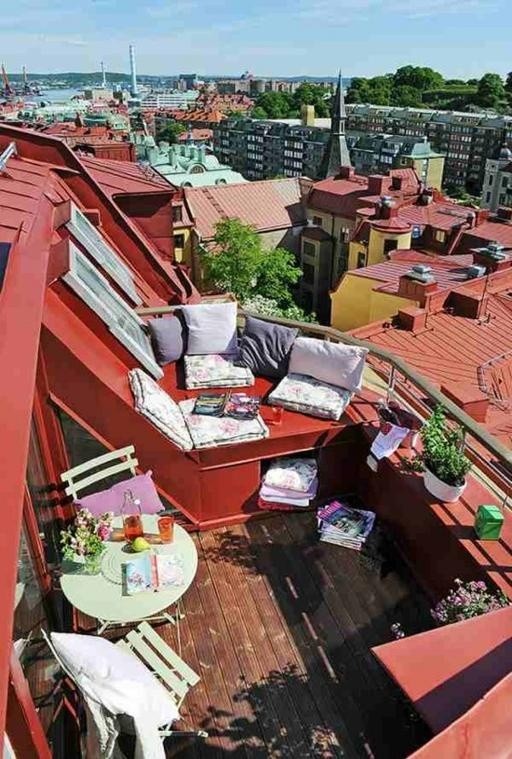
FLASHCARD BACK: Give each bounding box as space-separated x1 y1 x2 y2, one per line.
389 578 506 635
59 509 113 572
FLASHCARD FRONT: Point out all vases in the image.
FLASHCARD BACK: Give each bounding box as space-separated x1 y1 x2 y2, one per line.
85 557 102 575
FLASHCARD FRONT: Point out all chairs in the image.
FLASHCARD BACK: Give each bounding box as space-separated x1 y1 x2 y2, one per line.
60 512 199 636
39 620 209 754
54 444 165 519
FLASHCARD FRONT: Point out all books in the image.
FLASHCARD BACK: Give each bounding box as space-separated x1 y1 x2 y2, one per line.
190 387 263 421
316 499 377 553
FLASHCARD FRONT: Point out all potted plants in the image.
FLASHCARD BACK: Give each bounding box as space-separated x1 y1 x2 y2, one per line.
403 399 475 503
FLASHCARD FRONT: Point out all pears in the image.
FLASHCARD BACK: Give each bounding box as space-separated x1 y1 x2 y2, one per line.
131 536 151 552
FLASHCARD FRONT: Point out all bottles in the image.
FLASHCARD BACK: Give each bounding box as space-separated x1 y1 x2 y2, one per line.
120 488 144 542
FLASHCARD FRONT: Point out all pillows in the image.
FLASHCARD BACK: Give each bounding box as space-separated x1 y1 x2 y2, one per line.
72 470 165 527
235 314 299 379
288 336 367 397
46 633 180 729
179 301 239 355
147 315 183 365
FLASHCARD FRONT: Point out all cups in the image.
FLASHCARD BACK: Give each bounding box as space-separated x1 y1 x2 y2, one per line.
156 512 175 544
